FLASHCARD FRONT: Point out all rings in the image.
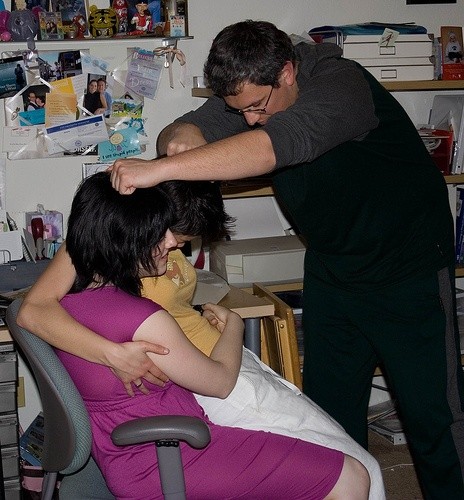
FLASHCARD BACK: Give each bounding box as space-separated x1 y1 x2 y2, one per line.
136 381 143 387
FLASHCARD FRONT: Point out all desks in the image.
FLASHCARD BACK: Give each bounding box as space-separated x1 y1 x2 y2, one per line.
0 285 275 361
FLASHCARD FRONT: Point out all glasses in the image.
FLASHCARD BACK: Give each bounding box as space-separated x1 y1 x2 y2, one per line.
224 85 275 116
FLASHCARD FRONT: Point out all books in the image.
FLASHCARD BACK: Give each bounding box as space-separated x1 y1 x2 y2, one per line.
447 184 464 267
456 288 464 354
275 294 304 373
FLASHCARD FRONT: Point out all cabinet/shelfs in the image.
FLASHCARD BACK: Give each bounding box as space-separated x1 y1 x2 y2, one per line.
192 80 464 199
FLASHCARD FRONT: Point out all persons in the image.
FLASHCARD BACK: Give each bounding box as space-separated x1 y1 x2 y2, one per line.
445 31 463 63
96 78 112 118
83 80 107 117
17 181 385 500
52 172 370 500
107 19 464 500
26 90 45 111
130 0 152 31
15 64 25 85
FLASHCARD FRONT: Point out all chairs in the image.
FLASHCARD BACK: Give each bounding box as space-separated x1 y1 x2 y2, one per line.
253 283 302 392
6 295 209 500
